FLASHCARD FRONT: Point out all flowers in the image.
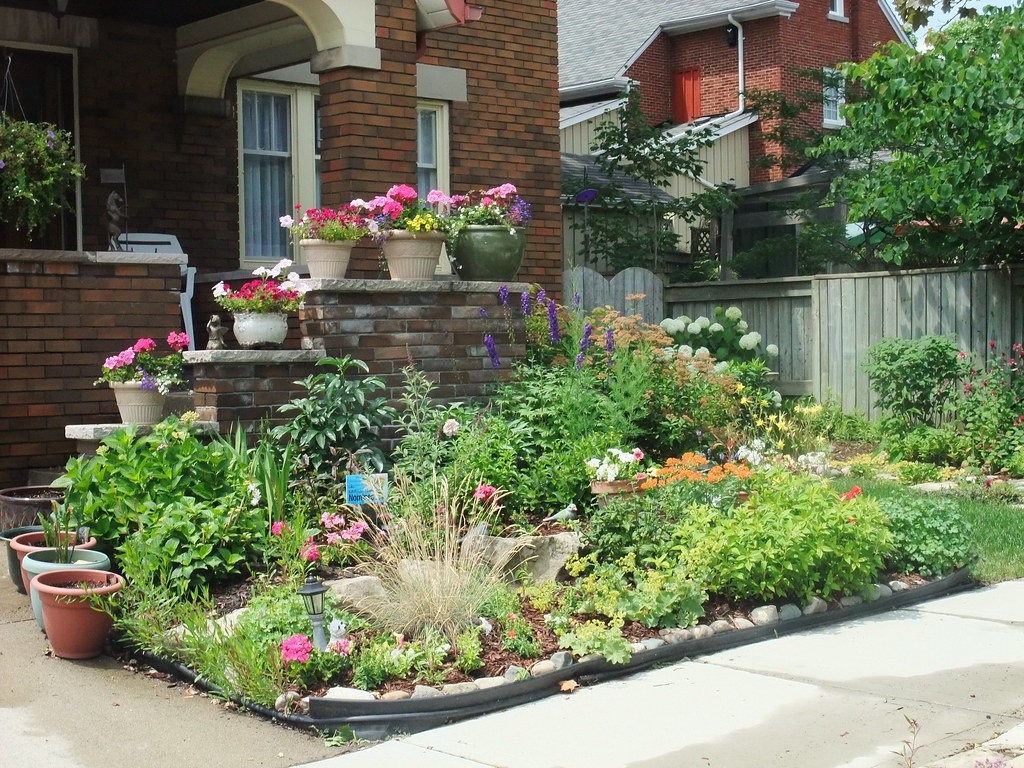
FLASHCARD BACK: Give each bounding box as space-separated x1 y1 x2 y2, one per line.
641 452 708 512
370 185 446 275
280 199 369 245
0 119 85 239
212 257 305 314
446 184 531 269
583 446 648 482
167 332 189 353
707 464 757 491
94 332 180 399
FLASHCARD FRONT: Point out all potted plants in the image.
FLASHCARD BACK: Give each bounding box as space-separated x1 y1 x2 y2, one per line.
21 486 111 633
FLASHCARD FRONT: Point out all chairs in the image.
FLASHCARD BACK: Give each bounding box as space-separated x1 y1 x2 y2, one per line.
108 234 196 351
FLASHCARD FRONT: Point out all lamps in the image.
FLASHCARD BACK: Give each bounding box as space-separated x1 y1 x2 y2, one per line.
296 577 331 621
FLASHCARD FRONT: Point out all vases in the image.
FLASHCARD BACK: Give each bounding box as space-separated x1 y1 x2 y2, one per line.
110 381 166 425
28 572 124 660
11 513 97 594
166 390 196 417
0 486 68 532
0 525 62 593
379 230 445 281
591 473 646 512
300 239 357 280
449 225 526 282
659 512 678 525
726 492 759 509
233 313 290 348
